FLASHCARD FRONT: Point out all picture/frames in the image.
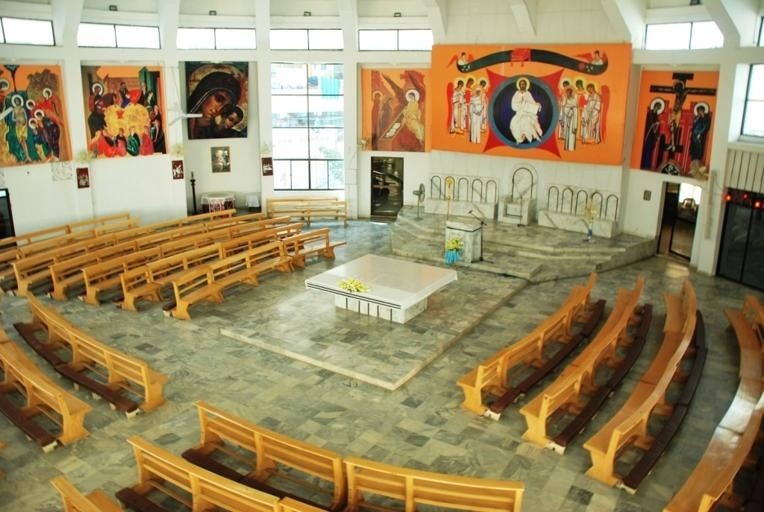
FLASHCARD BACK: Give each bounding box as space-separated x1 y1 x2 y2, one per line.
208 144 232 174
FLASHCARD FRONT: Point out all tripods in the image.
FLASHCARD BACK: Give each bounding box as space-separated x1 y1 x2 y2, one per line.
471 213 494 264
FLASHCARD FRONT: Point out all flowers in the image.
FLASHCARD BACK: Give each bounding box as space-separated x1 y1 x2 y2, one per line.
338 278 370 294
445 235 465 254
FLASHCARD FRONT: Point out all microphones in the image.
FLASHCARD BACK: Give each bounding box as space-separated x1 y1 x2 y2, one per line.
468 210 473 213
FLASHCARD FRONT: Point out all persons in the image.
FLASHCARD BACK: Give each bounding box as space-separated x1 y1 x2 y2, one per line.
217 106 244 136
191 73 246 136
84 78 167 156
450 50 612 155
403 90 425 148
1 77 67 163
645 82 708 177
369 87 382 141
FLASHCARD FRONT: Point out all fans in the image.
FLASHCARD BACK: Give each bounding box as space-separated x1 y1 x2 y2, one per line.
412 183 426 222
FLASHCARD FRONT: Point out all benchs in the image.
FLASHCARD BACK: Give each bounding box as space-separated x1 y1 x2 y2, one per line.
454 273 763 512
0 192 351 322
2 291 528 512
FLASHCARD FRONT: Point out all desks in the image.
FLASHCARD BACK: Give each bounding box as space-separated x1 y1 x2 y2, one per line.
305 253 457 324
